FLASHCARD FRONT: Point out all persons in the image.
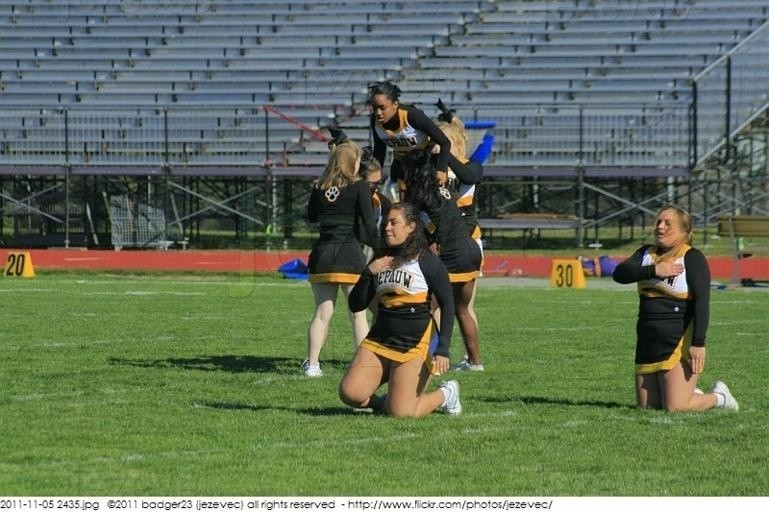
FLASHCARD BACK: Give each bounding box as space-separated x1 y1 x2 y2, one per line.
614 205 739 413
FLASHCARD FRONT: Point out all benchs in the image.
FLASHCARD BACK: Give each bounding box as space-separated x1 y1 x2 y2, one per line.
2 0 768 161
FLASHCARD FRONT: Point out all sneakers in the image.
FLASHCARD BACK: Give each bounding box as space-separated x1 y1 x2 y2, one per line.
441 380 462 415
302 363 322 376
452 360 485 372
694 380 739 412
